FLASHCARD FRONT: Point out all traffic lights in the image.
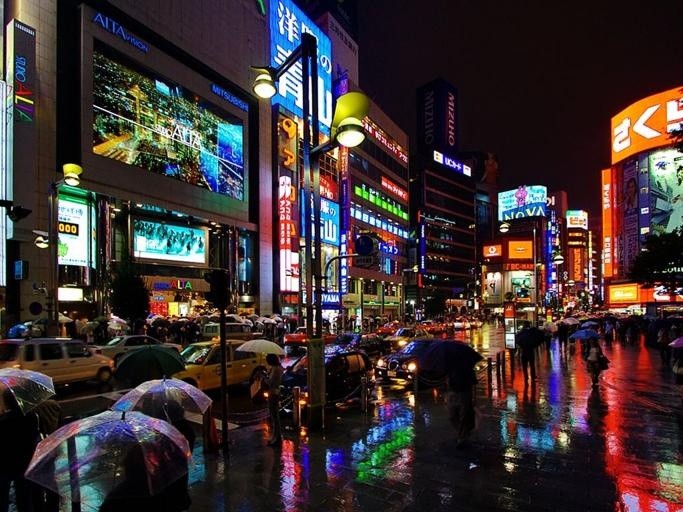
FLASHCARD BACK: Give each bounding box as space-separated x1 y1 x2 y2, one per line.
203 267 231 309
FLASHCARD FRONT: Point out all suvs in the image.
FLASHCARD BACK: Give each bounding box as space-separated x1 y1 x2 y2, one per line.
203 323 264 340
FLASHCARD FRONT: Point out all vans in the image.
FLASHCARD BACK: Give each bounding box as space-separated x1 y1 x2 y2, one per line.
0 337 115 389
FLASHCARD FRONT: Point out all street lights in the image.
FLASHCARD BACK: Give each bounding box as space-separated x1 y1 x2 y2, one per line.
32 162 82 338
499 218 564 329
395 265 419 324
250 33 368 429
537 254 576 314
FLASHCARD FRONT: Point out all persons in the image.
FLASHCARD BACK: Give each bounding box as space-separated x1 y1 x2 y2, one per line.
515 315 618 383
252 308 304 341
259 354 284 446
98 397 197 510
23 313 203 349
617 310 683 385
565 309 571 318
338 310 505 334
2 386 62 511
446 373 478 437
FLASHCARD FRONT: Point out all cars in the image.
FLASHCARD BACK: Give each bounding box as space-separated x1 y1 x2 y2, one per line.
263 349 375 416
165 335 280 399
323 332 391 359
83 335 183 366
375 340 482 387
283 326 336 343
337 311 547 352
